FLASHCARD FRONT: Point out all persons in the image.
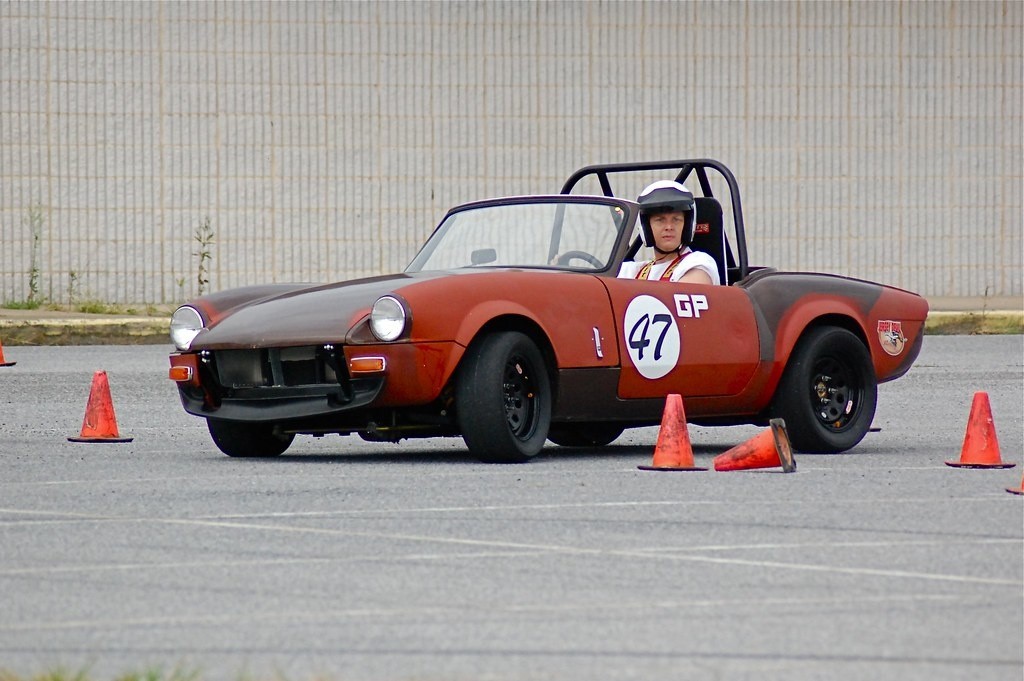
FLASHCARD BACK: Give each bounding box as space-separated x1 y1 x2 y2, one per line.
550 180 721 285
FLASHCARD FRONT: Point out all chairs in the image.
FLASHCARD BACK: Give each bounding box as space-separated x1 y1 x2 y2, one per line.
689 197 728 286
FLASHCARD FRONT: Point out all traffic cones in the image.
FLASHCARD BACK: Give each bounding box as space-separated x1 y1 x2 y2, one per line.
944 392 1016 469
0 337 17 367
713 417 798 473
637 392 710 473
66 371 134 445
1005 471 1024 496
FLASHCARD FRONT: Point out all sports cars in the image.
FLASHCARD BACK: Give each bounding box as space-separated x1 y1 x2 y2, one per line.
167 156 932 466
